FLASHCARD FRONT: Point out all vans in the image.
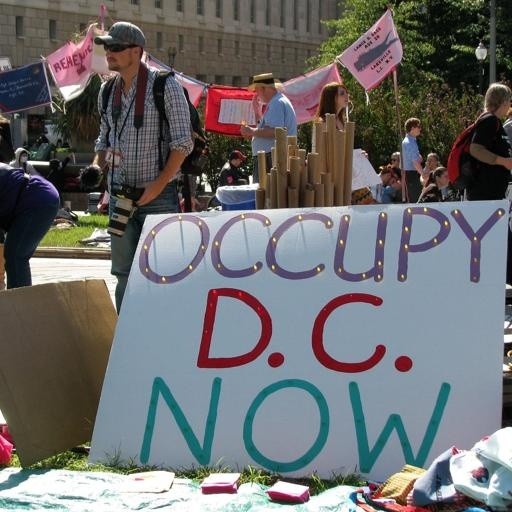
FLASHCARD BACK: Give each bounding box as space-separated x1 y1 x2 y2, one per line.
43 124 64 149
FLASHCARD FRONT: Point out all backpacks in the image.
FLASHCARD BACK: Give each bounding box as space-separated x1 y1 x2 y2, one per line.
447 111 499 187
102 70 210 177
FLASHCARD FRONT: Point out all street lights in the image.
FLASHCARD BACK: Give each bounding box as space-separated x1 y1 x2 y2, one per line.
473 0 497 87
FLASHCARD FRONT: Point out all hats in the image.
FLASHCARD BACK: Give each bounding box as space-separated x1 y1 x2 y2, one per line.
248 73 276 88
229 151 248 160
94 22 146 48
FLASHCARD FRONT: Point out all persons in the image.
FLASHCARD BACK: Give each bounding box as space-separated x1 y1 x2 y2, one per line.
0 163 60 288
9 148 39 176
502 107 512 290
218 150 247 187
240 72 297 183
312 81 349 155
371 118 451 203
92 21 195 317
446 82 512 200
180 88 206 211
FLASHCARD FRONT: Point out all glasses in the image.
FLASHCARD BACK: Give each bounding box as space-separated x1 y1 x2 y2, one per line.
103 42 131 52
